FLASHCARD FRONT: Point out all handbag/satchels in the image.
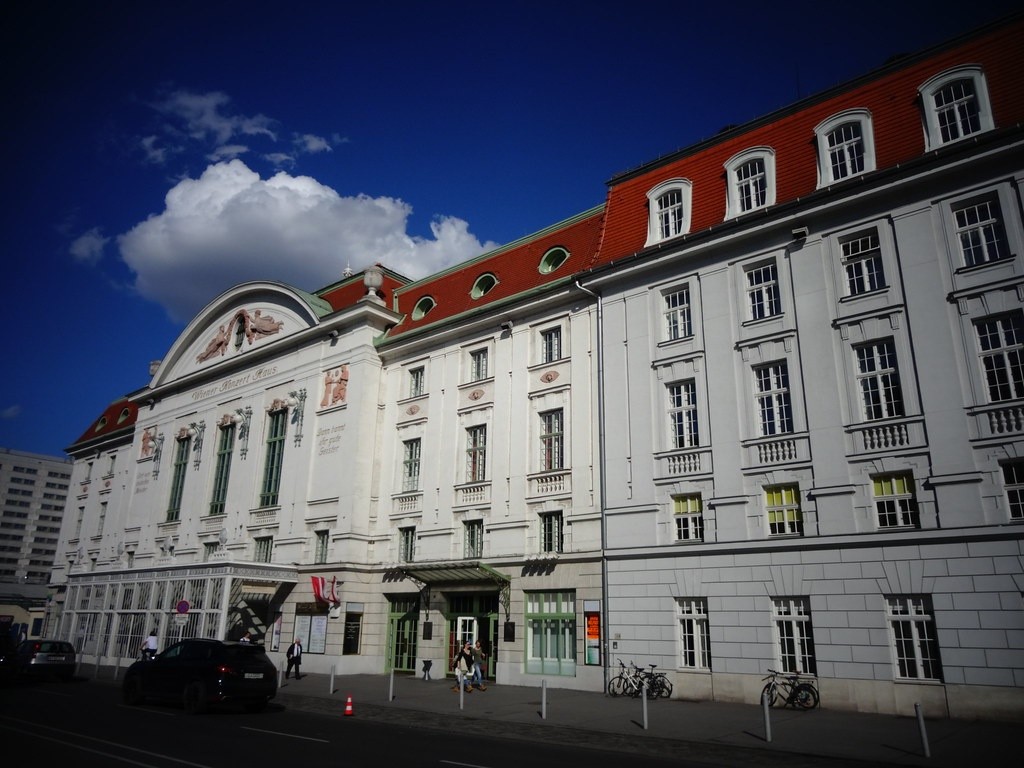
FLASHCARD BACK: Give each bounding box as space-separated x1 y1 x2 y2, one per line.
455 667 467 682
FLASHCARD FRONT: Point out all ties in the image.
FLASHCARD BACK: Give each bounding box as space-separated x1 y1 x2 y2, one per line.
297 645 299 657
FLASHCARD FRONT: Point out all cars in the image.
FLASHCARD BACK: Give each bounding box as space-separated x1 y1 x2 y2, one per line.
7 640 76 682
121 638 277 715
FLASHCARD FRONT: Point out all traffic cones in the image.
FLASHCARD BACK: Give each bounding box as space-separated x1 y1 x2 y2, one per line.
344 695 353 716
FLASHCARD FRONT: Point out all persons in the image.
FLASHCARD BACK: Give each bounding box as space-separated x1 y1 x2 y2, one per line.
246 310 284 335
196 326 228 364
333 365 348 404
320 371 334 406
454 640 488 692
240 631 251 642
139 631 158 660
141 428 151 458
285 639 302 680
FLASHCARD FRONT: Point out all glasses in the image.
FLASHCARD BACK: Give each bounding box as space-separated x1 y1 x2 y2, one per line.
466 644 470 646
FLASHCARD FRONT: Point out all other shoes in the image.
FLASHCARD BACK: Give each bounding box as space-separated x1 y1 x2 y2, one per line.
295 678 301 680
466 685 473 693
286 674 289 679
454 686 460 693
477 685 487 691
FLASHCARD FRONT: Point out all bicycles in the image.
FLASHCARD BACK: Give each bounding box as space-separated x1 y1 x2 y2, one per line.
762 668 818 710
135 648 157 662
608 658 674 700
